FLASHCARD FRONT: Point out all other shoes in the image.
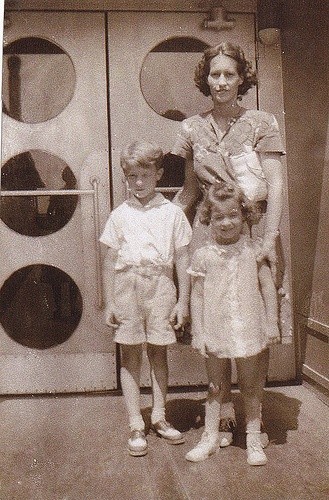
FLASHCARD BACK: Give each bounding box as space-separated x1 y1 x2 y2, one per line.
245 430 266 466
260 422 268 448
218 415 237 447
185 432 219 462
127 422 147 456
149 420 184 445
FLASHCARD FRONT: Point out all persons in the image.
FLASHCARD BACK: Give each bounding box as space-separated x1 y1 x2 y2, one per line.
186 180 281 465
98 142 191 456
162 41 286 449
